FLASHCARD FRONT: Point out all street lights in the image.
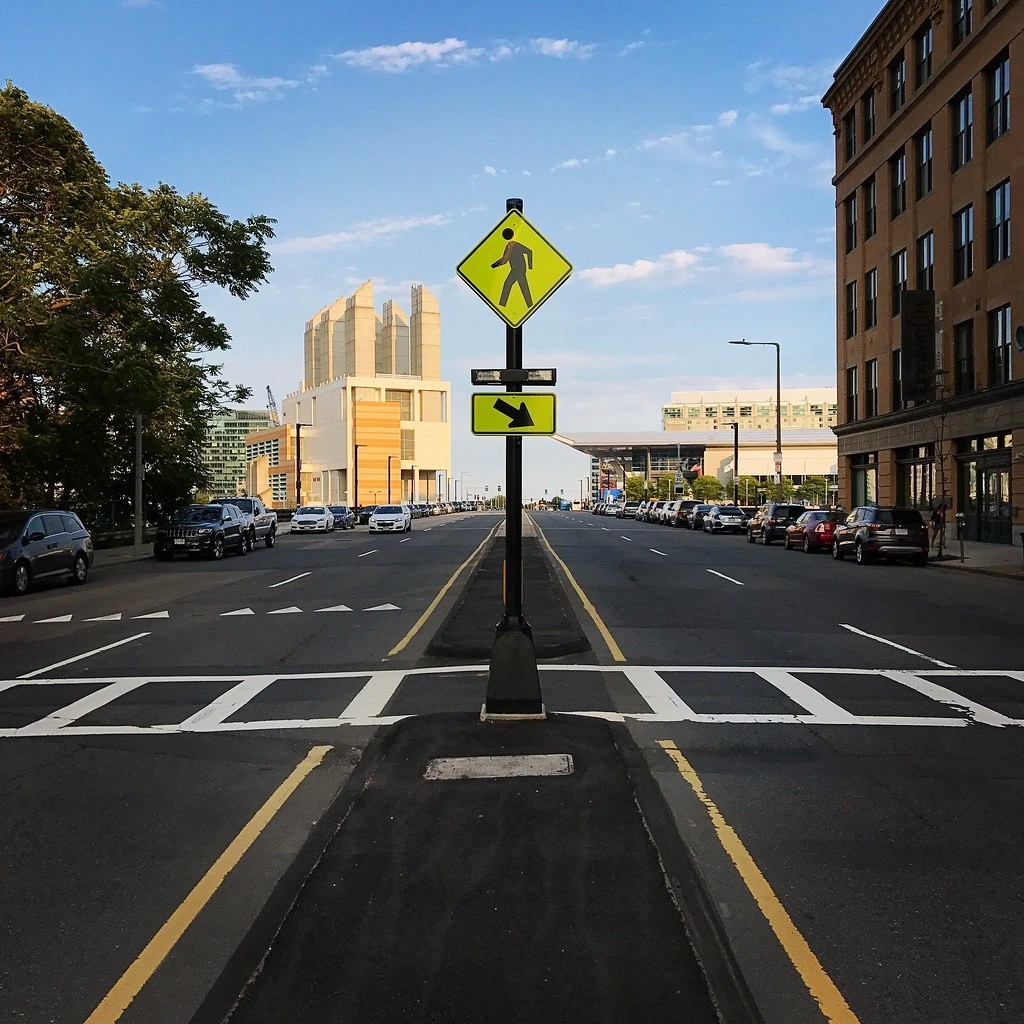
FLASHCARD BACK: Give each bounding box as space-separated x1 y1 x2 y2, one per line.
619 464 625 501
388 455 399 504
355 444 366 507
729 339 782 503
412 465 419 504
369 491 382 504
722 422 738 507
296 423 313 508
639 461 647 502
427 471 460 504
585 476 589 510
579 480 582 510
607 470 609 504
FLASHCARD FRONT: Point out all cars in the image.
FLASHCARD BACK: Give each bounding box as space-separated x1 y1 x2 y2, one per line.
701 506 747 534
289 505 335 533
783 511 850 553
591 503 616 516
369 504 412 533
485 506 506 511
359 500 478 524
686 504 717 529
326 506 357 530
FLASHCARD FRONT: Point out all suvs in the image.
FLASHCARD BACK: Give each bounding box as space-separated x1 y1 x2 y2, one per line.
746 504 806 545
668 500 704 527
616 501 640 519
635 500 654 522
154 503 248 560
831 505 929 567
0 506 95 594
648 501 666 522
659 501 676 525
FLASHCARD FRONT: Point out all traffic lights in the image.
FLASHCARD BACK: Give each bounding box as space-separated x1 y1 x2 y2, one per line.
561 489 564 495
545 489 548 496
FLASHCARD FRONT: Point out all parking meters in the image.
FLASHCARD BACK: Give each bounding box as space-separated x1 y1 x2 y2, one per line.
955 513 966 563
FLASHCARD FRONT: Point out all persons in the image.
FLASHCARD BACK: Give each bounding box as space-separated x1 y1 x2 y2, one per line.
930 503 947 549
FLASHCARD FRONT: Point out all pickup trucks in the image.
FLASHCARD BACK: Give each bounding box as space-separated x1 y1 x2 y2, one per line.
210 496 279 552
